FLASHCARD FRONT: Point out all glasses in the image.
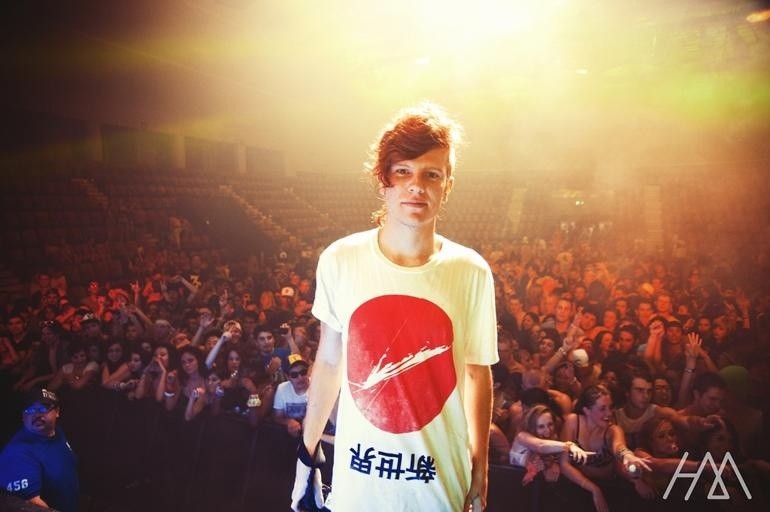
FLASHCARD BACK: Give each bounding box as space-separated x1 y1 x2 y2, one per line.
22 404 51 416
654 384 673 393
290 368 309 380
716 433 737 445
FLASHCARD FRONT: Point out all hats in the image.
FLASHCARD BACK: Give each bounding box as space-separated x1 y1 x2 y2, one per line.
286 354 309 370
23 384 61 406
281 286 295 297
643 282 655 297
109 287 130 300
569 349 589 367
224 320 243 332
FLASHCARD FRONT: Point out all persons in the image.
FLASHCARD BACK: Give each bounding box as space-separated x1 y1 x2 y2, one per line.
292 105 499 512
